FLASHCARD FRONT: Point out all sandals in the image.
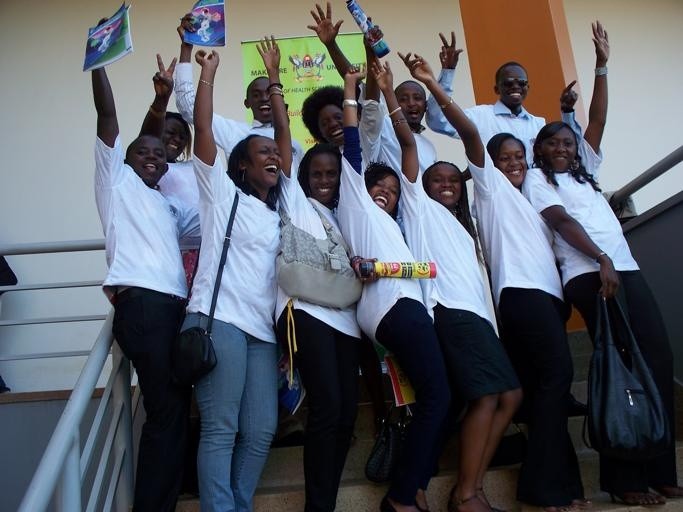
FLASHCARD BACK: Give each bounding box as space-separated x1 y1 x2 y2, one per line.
609 482 682 506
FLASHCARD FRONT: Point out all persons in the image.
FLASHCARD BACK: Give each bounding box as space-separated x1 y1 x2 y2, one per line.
82 1 302 510
427 22 682 504
256 0 449 511
372 53 596 511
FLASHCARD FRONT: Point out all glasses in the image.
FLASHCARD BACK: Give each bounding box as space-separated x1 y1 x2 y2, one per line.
500 77 529 87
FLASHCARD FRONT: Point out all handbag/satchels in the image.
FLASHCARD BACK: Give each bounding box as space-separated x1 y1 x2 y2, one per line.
586 333 667 454
364 417 406 485
157 325 218 389
277 219 364 312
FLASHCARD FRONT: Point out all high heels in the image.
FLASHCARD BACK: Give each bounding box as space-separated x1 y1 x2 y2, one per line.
379 488 432 512
447 484 503 512
